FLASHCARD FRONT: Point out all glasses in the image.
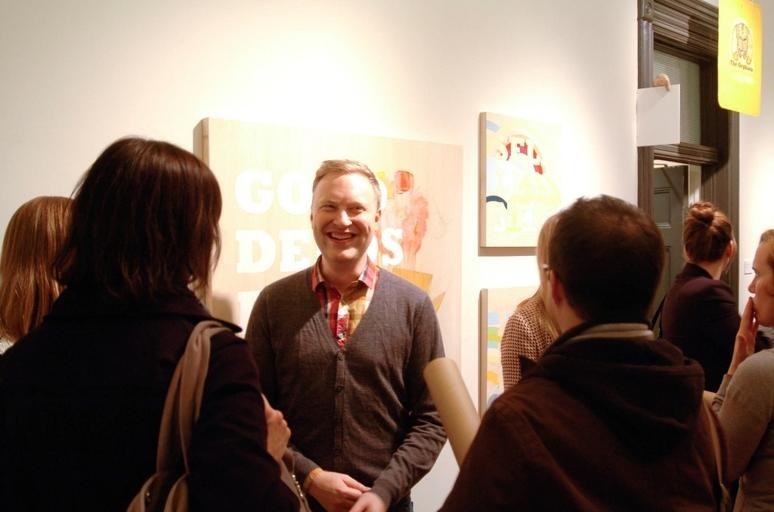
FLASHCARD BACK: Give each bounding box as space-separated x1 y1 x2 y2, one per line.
543 264 556 277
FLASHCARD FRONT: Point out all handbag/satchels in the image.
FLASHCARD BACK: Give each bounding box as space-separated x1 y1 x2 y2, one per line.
126 321 311 510
650 296 665 330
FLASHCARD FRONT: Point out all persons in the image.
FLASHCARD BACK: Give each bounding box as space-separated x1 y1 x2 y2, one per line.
246 158 446 512
660 199 770 393
710 228 774 512
2 137 300 511
500 211 565 394
1 196 74 351
439 195 729 511
